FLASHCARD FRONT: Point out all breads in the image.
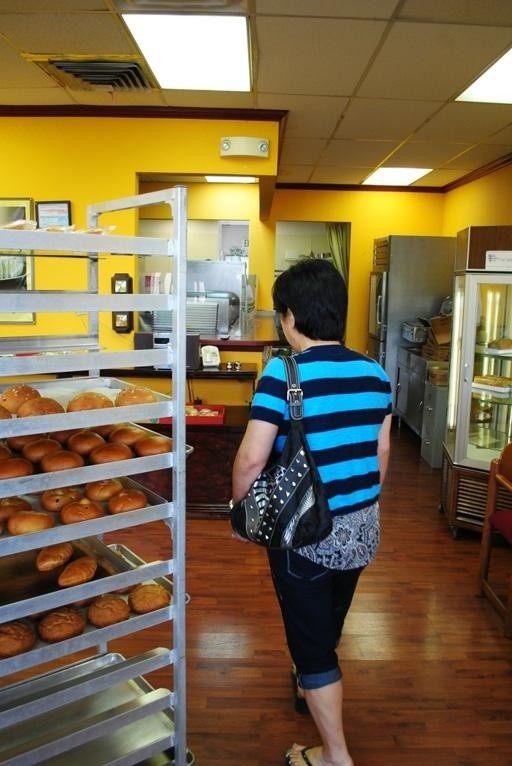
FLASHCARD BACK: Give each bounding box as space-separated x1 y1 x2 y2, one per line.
0 424 172 480
33 541 97 589
0 380 157 419
0 580 173 658
0 480 148 536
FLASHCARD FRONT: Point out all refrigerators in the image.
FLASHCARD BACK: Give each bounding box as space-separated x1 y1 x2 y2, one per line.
365 235 457 416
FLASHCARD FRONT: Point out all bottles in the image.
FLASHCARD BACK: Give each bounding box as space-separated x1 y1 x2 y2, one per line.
477 401 493 424
474 315 487 344
478 422 491 440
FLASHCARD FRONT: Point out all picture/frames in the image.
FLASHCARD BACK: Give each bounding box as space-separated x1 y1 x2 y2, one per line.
0 196 36 326
34 199 73 232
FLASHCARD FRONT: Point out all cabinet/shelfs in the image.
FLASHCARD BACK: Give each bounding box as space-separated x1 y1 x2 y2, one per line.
434 444 510 542
418 381 448 469
394 346 449 439
437 272 512 474
1 183 201 763
55 360 260 520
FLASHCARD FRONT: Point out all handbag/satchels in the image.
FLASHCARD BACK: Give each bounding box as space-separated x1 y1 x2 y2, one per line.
229 403 333 550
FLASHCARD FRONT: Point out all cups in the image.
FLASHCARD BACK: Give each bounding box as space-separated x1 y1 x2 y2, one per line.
146 272 204 297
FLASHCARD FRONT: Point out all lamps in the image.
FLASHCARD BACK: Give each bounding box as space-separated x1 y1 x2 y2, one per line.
219 134 271 161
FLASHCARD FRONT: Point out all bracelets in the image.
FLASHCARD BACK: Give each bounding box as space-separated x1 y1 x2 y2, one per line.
227 496 234 513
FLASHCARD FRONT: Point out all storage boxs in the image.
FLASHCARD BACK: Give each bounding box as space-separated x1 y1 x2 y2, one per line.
421 314 452 386
452 224 512 274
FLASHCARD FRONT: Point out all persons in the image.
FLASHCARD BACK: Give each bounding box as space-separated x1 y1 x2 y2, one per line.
226 256 393 766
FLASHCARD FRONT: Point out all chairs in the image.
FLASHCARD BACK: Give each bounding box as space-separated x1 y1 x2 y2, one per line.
476 442 511 640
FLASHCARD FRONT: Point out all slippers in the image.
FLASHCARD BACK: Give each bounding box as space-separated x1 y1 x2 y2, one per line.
284 743 321 766
290 662 311 716
